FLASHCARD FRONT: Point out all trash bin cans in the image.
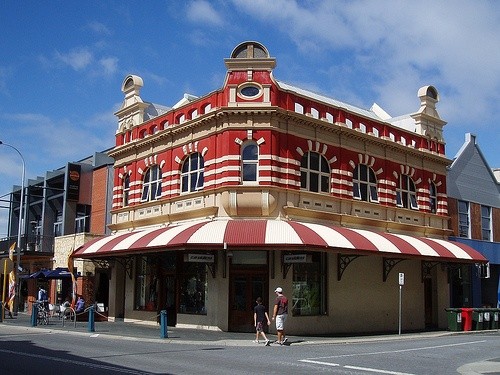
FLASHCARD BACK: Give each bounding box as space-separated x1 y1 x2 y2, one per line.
473 307 483 330
459 307 473 330
478 307 490 329
488 307 499 328
447 307 463 331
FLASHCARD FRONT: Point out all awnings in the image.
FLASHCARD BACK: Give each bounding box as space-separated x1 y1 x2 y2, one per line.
70 218 489 265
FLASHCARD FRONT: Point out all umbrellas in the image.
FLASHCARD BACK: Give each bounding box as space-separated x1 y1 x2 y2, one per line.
29 269 81 304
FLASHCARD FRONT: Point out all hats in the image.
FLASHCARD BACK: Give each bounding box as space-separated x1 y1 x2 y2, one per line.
257 297 263 302
274 287 283 292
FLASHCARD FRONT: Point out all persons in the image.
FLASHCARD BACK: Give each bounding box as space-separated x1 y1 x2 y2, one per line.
65 295 85 315
270 287 288 345
253 297 272 347
38 285 49 315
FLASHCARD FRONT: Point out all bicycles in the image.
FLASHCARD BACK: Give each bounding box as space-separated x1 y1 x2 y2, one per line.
32 301 49 325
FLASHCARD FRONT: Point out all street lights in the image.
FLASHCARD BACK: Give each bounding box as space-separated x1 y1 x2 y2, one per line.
0 140 26 316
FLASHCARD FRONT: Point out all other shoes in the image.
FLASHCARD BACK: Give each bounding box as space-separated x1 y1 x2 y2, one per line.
275 342 281 345
253 340 259 344
282 338 288 344
265 340 270 346
